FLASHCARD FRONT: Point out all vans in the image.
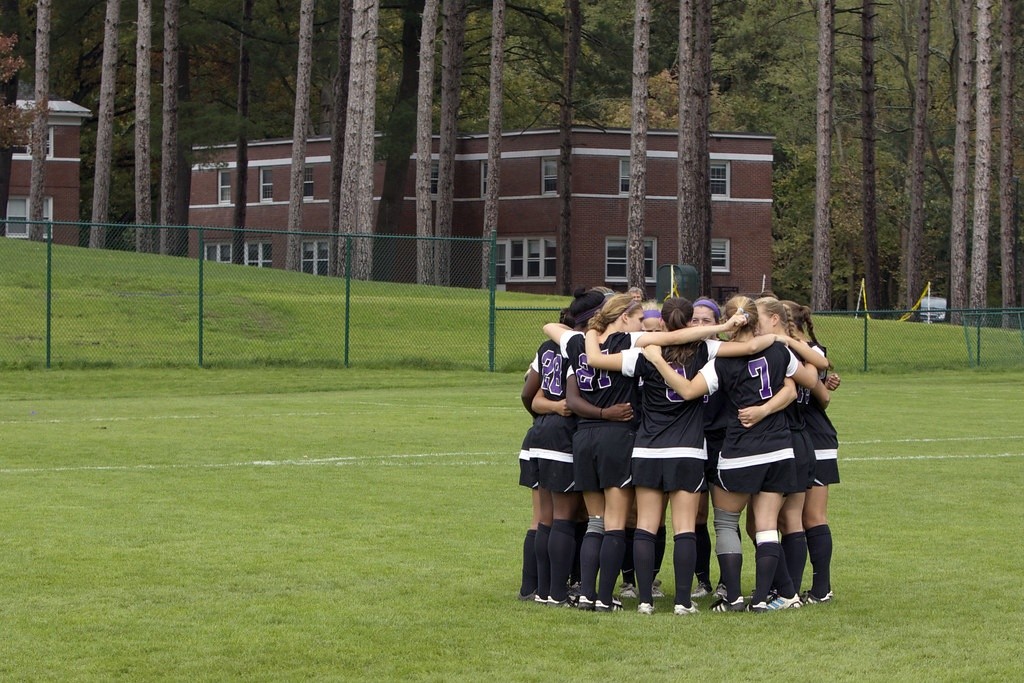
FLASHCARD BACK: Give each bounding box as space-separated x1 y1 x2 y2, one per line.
920 296 951 323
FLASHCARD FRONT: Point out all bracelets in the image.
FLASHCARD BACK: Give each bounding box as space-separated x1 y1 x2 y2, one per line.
599 407 604 420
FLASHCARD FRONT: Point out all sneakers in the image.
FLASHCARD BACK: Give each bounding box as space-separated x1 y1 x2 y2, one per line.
568 580 581 591
547 593 574 608
691 581 714 605
652 579 665 598
767 592 804 611
534 594 547 605
596 600 624 613
799 588 834 605
712 582 727 600
745 600 768 612
637 603 656 616
709 595 745 613
765 590 777 603
565 585 580 600
577 595 595 610
744 590 757 599
618 582 637 598
518 588 537 602
674 601 700 616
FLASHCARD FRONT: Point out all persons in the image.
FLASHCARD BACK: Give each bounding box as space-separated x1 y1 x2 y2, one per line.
518 288 841 612
